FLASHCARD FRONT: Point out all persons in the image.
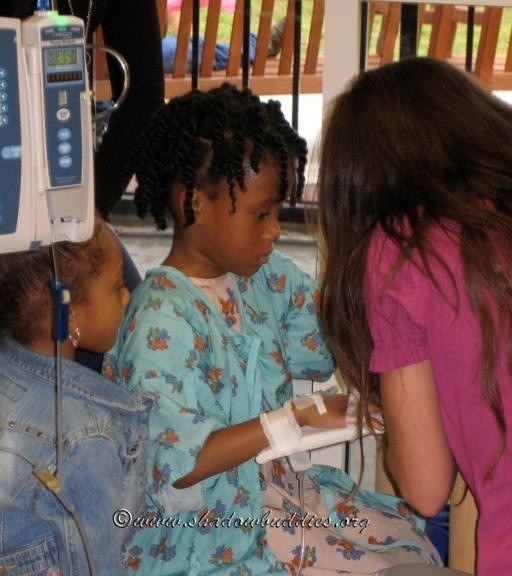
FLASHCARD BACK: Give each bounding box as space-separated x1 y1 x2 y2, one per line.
311 57 512 575
93 1 170 231
162 15 287 72
0 210 153 576
114 82 468 575
